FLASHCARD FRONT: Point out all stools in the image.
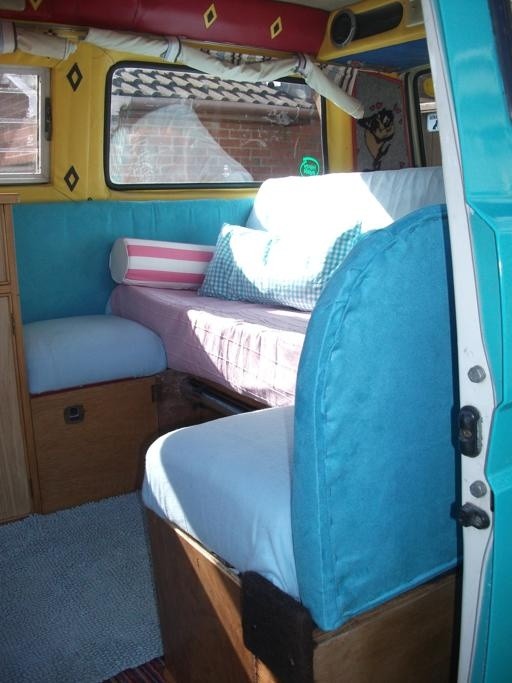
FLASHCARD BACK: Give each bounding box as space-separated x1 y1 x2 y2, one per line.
22 315 165 514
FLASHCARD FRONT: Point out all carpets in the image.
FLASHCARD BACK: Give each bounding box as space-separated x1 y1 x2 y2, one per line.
1 488 164 683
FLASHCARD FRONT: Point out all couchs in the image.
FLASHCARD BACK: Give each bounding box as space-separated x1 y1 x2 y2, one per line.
108 167 446 406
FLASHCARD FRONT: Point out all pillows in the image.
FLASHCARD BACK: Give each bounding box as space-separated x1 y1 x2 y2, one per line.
200 225 362 312
109 237 215 290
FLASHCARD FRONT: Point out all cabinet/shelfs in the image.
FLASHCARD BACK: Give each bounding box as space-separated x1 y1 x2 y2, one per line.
0 194 32 526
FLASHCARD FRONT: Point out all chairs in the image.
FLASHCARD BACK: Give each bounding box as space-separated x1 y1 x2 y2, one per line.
145 205 463 683
